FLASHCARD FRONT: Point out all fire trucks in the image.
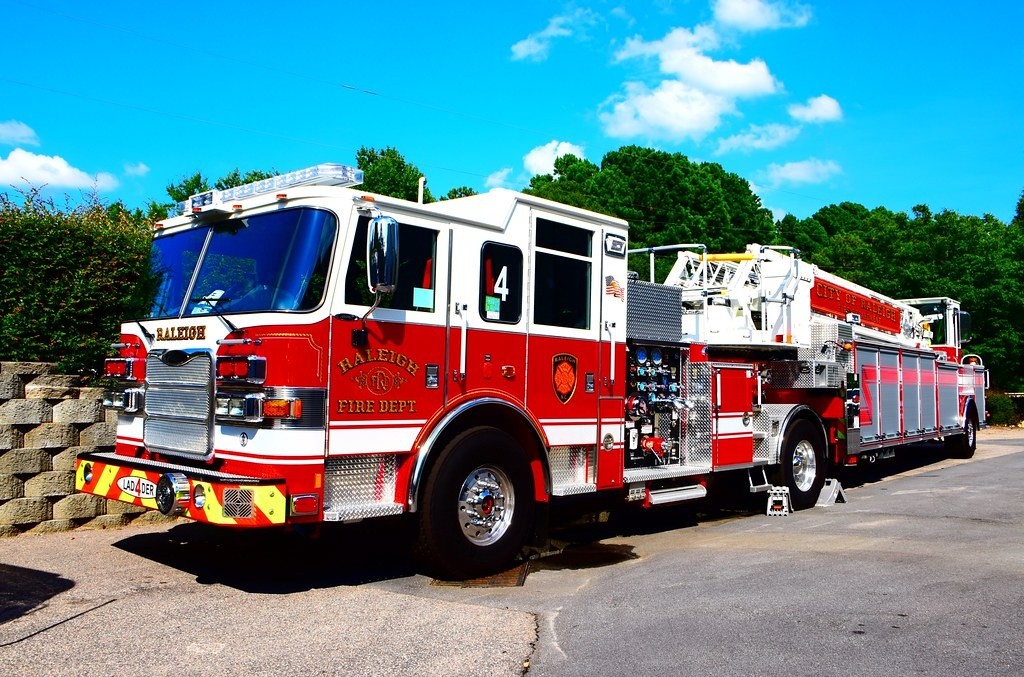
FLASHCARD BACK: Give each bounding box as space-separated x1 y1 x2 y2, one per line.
74 163 990 582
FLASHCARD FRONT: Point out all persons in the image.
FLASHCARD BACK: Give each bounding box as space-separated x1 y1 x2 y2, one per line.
218 259 363 311
